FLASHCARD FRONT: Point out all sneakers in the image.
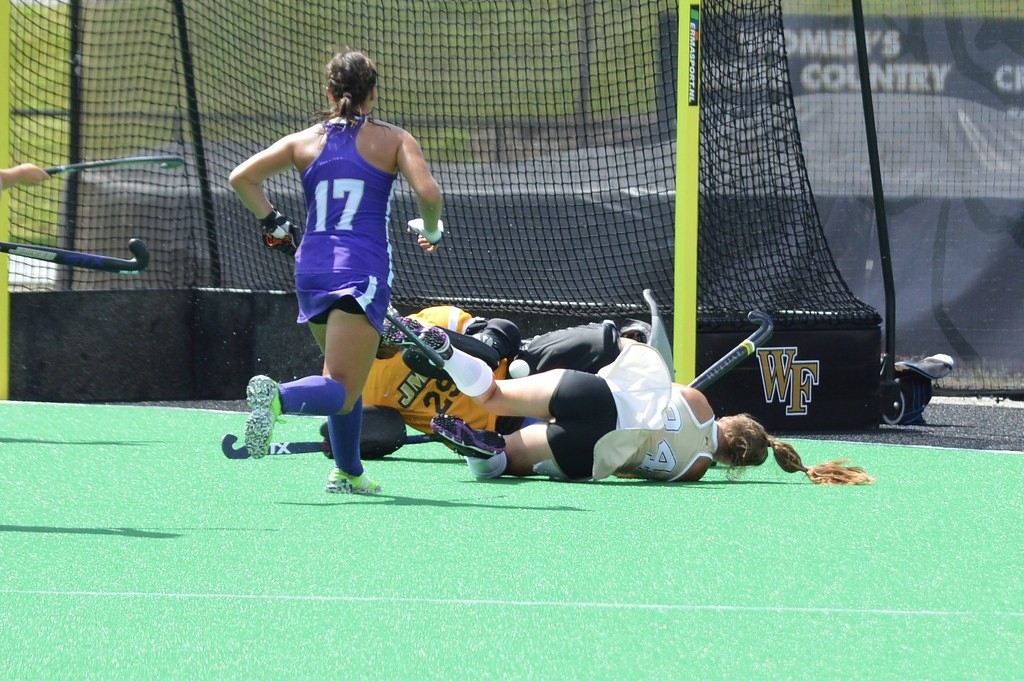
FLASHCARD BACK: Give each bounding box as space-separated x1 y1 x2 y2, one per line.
430 413 506 460
244 375 287 459
382 316 454 361
324 460 382 494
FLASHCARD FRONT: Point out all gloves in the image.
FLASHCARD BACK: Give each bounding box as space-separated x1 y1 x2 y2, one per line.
257 205 302 257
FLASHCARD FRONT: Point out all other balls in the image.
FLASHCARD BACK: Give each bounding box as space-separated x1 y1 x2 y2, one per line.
508 359 531 379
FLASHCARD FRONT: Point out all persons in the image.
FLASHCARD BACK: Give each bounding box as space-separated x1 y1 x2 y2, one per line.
325 305 875 486
0 163 51 188
230 50 443 493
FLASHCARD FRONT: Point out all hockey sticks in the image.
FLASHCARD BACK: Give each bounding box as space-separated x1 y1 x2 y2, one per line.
220 433 436 460
686 310 774 393
264 220 445 368
